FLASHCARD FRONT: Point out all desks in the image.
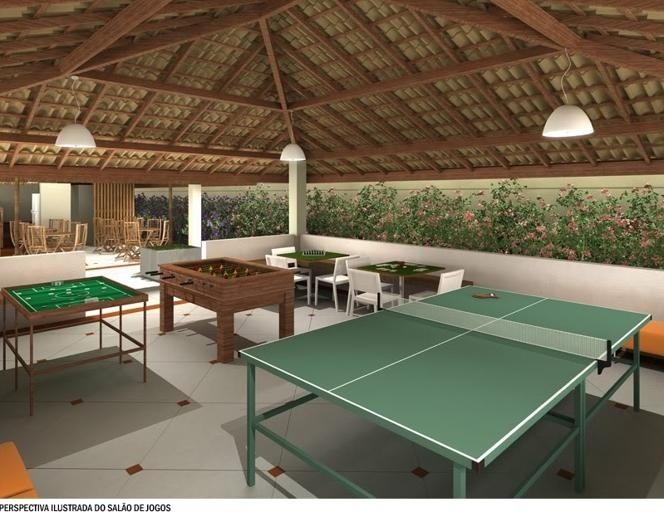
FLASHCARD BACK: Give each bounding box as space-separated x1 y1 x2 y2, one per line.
234 282 656 499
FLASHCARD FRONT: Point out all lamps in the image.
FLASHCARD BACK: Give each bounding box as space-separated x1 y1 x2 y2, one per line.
51 74 97 150
278 109 306 163
538 44 595 139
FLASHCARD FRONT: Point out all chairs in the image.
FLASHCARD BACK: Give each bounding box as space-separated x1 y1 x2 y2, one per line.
7 214 170 268
259 241 467 319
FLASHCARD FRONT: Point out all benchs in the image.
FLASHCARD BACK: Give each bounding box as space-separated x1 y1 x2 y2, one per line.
618 315 663 363
